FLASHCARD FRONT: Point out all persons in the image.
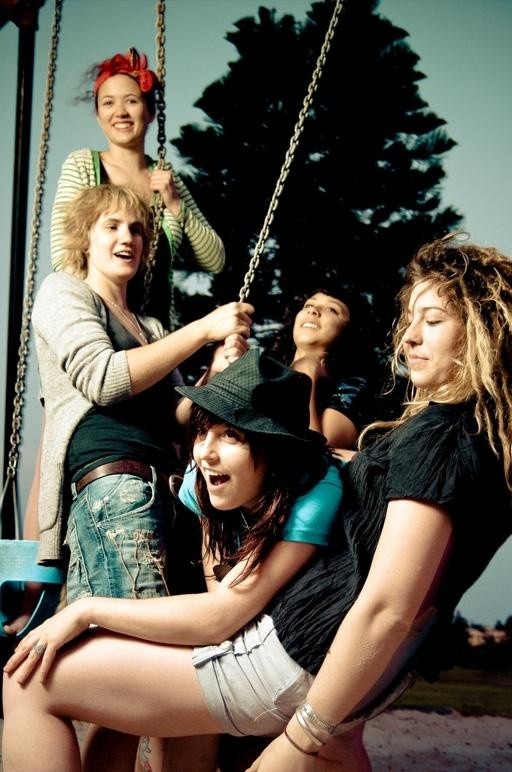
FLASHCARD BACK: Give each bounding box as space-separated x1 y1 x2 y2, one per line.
49 47 227 332
31 182 258 772
0 227 512 772
2 344 352 689
172 283 406 451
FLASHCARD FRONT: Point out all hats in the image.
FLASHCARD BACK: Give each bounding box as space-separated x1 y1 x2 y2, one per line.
169 342 339 456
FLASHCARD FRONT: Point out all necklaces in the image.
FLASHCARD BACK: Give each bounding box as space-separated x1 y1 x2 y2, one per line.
94 290 149 346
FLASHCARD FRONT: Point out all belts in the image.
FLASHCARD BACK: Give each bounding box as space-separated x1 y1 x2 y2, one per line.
64 460 173 503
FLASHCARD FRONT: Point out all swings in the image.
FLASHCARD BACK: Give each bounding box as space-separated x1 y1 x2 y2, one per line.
0 1 168 582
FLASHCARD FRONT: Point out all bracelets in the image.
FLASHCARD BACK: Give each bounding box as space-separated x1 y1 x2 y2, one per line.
295 702 335 746
279 728 325 758
